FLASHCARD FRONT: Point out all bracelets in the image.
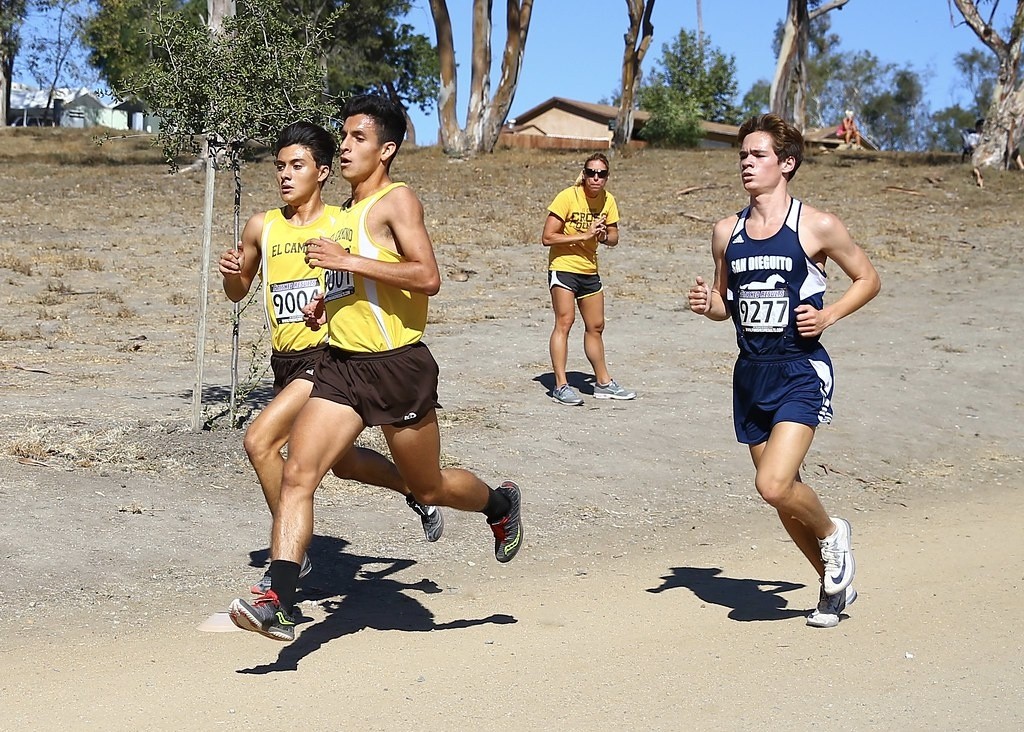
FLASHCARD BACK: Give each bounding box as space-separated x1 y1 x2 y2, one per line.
596 232 609 243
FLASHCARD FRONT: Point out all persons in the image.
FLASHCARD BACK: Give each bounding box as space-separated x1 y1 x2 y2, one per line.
687 112 885 631
217 124 444 597
540 152 639 407
836 109 861 146
226 91 526 644
967 118 1024 173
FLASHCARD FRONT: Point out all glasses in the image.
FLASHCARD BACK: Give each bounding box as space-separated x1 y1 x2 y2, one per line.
584 167 608 178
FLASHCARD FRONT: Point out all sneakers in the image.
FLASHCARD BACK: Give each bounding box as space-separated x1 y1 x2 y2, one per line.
406 496 444 542
552 384 584 406
816 518 854 595
227 590 301 641
251 552 312 595
593 379 636 399
806 578 857 628
486 481 523 563
